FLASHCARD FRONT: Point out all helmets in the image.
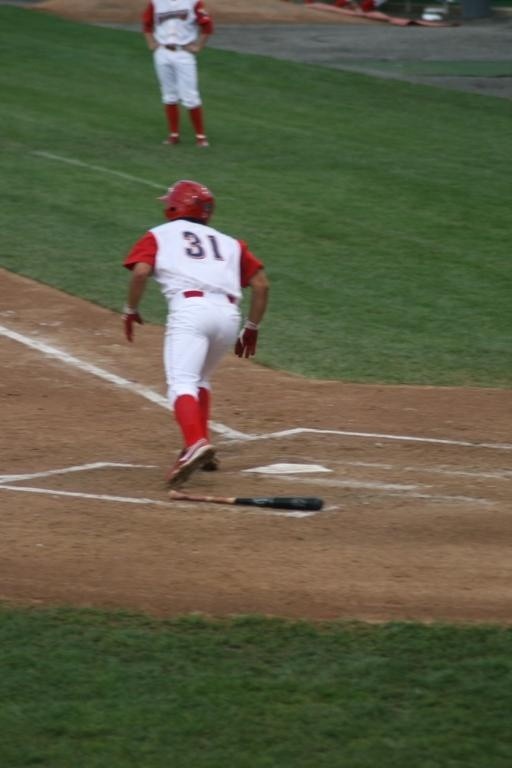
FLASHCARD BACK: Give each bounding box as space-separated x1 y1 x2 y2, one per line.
156 179 216 224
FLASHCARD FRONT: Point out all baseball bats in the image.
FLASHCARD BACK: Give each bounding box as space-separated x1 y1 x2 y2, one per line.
169 489 322 511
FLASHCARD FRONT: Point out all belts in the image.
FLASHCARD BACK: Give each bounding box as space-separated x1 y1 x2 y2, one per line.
182 289 238 304
164 44 184 51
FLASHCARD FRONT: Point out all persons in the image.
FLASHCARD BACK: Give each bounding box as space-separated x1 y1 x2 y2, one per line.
140 1 214 146
121 174 269 483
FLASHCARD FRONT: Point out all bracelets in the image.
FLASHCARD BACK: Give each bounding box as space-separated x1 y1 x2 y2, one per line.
126 305 139 315
244 316 259 331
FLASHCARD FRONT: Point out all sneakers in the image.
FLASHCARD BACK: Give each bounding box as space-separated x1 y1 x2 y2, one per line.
195 134 208 147
166 437 217 486
163 132 181 146
201 455 221 472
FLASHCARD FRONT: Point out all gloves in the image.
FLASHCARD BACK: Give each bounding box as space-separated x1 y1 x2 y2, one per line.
235 320 261 360
122 306 143 343
